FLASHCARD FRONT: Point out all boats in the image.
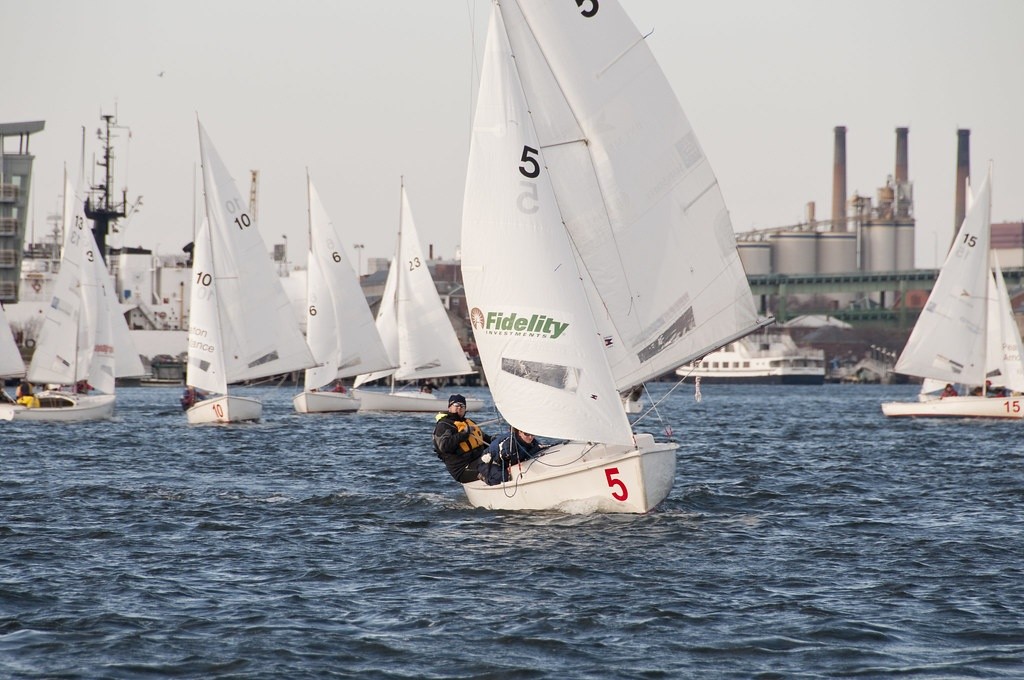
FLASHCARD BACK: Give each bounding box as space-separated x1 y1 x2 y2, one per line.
676 326 828 385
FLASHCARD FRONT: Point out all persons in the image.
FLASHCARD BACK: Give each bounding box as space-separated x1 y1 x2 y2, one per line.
76 378 96 395
180 383 209 411
15 376 39 409
432 394 496 483
332 381 347 395
477 426 545 488
421 380 439 395
968 378 991 397
939 383 957 397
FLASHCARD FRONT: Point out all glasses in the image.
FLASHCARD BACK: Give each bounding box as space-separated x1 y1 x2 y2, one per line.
521 432 534 436
451 402 465 408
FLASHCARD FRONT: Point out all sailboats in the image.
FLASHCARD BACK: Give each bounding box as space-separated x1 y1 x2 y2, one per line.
291 165 401 415
181 111 321 424
0 159 151 423
882 160 1024 419
350 174 486 413
460 0 777 517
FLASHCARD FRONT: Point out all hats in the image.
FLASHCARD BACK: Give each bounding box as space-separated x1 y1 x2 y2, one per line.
448 394 466 408
